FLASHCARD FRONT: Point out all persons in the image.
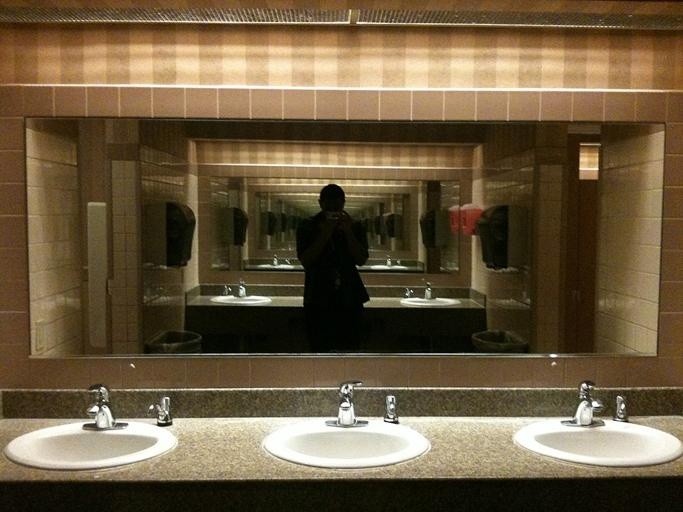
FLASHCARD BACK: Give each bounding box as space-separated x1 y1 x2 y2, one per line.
294 184 370 353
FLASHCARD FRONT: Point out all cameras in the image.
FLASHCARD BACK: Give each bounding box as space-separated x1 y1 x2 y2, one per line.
328 211 342 220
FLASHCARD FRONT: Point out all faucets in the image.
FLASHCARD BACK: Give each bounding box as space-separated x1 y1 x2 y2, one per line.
574 380 605 424
425 282 433 300
383 396 400 423
614 394 629 418
86 385 115 429
337 381 361 426
239 284 246 297
146 397 171 425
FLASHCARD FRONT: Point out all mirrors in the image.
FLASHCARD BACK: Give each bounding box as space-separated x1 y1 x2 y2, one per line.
24 116 666 358
241 180 428 268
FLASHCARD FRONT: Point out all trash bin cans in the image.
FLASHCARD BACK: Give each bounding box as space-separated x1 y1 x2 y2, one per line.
472 328 527 353
148 330 201 354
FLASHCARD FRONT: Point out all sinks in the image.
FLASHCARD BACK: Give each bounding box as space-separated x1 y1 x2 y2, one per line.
264 418 429 469
3 421 174 470
211 295 271 305
514 419 679 466
400 296 460 306
